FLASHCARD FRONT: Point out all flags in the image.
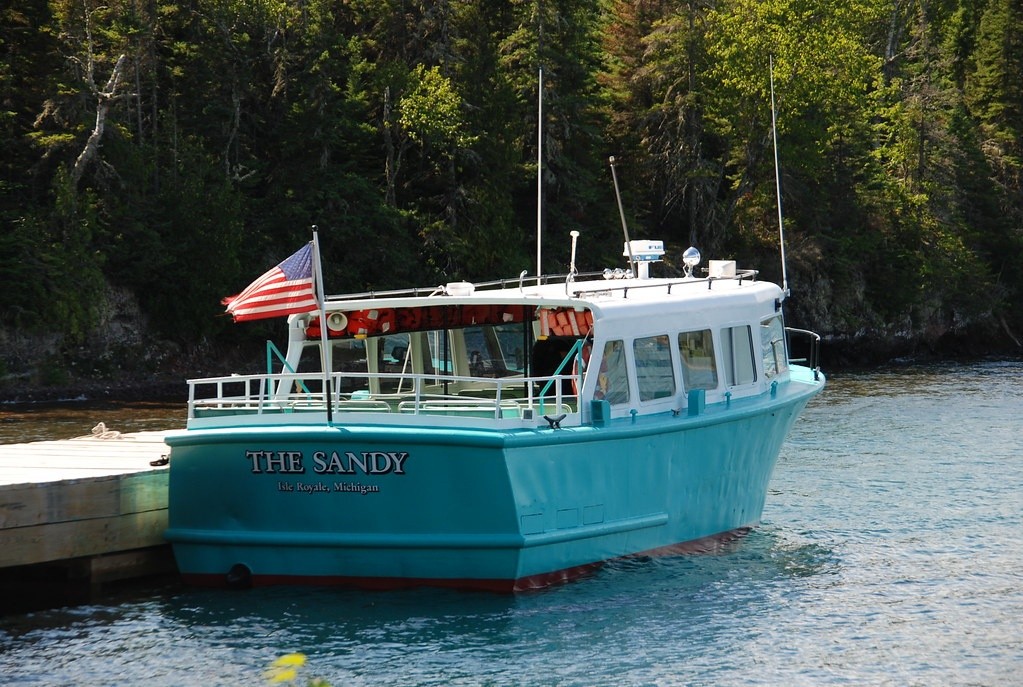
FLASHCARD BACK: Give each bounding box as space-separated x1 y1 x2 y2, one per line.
221 240 319 323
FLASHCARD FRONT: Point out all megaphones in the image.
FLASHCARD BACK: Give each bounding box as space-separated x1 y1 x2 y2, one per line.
327 312 348 331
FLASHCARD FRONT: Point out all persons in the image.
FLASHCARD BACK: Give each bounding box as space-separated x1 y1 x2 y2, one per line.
654 335 675 398
470 351 497 378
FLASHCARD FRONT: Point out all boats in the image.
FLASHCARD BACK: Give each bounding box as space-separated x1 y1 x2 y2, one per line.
166 54 827 590
